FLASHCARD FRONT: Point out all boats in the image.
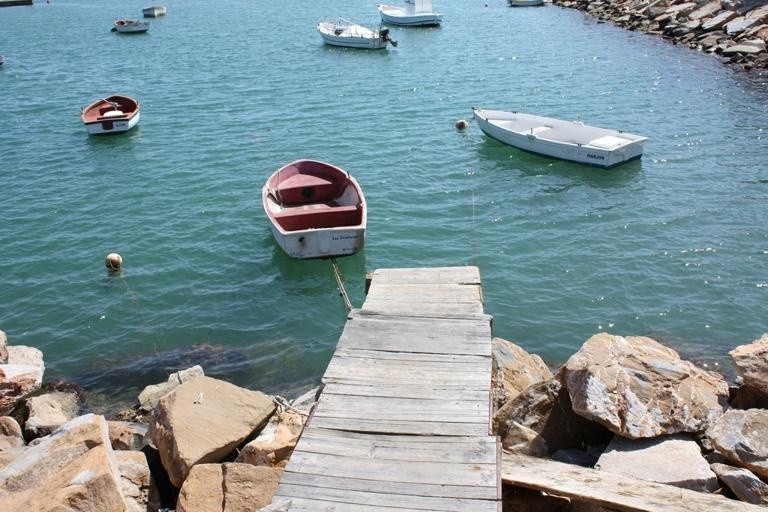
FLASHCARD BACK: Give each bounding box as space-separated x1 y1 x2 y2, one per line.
317 17 396 51
507 0 544 8
264 159 369 263
469 104 646 170
0 55 4 65
112 17 151 34
142 5 166 19
80 91 142 136
377 0 442 27
